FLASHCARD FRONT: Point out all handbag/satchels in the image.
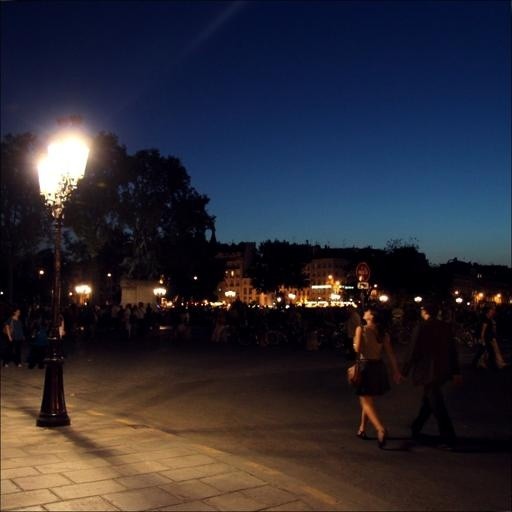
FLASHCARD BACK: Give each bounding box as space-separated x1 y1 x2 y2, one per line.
345 361 364 386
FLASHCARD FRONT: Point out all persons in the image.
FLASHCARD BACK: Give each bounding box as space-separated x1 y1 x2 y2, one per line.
397 302 464 453
351 306 400 449
1 295 511 372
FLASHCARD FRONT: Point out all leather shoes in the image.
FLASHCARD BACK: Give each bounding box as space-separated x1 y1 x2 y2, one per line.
356 428 461 452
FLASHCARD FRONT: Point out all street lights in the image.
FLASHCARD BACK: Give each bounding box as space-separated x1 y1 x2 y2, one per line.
37 126 90 427
